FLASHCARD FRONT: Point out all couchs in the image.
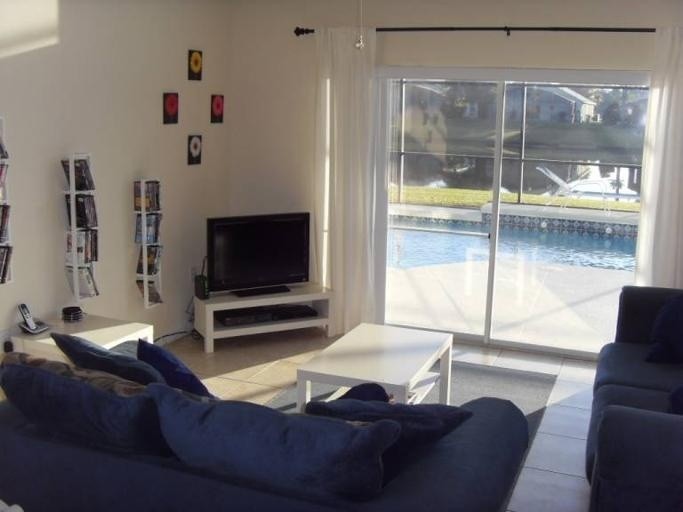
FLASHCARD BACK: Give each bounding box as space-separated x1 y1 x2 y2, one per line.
0 352 528 512
586 285 682 510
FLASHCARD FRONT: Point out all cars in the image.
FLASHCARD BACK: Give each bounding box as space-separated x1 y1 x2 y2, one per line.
545 177 639 202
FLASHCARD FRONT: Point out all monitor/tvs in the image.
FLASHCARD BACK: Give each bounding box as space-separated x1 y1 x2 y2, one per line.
205 211 310 297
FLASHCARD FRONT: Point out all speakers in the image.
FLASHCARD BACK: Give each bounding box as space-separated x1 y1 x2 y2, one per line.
194 275 208 300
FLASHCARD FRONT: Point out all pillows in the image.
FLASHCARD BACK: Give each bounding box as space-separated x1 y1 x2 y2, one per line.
641 291 681 365
51 331 212 398
301 398 472 456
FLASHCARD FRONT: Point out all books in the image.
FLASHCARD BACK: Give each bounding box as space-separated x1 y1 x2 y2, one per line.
60 155 101 303
0 138 13 285
132 177 163 305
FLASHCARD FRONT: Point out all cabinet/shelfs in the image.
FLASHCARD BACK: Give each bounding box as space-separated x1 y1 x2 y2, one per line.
192 283 336 354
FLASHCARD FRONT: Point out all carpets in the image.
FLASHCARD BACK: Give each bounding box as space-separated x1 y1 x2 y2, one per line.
257 356 557 509
